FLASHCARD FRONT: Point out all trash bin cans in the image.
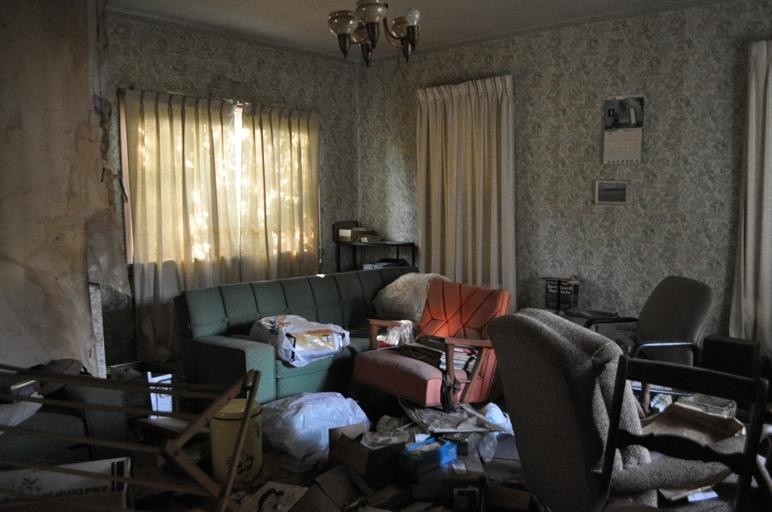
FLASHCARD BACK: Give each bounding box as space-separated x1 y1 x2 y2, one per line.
209 398 263 483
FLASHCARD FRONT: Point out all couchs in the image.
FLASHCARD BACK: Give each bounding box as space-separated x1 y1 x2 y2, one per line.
173 265 421 404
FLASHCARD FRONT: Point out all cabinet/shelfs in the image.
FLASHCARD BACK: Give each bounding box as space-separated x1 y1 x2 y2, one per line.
0 363 261 512
336 241 415 272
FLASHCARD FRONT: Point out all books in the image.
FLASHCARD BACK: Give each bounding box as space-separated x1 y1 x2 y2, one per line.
397 329 480 376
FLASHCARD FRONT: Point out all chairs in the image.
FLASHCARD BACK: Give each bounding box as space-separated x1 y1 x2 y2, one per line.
583 276 715 395
486 307 771 511
354 278 511 408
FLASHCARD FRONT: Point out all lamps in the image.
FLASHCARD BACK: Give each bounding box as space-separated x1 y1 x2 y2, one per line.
328 0 421 67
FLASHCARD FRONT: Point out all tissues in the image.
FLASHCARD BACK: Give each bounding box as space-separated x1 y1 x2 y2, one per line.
400 437 457 481
